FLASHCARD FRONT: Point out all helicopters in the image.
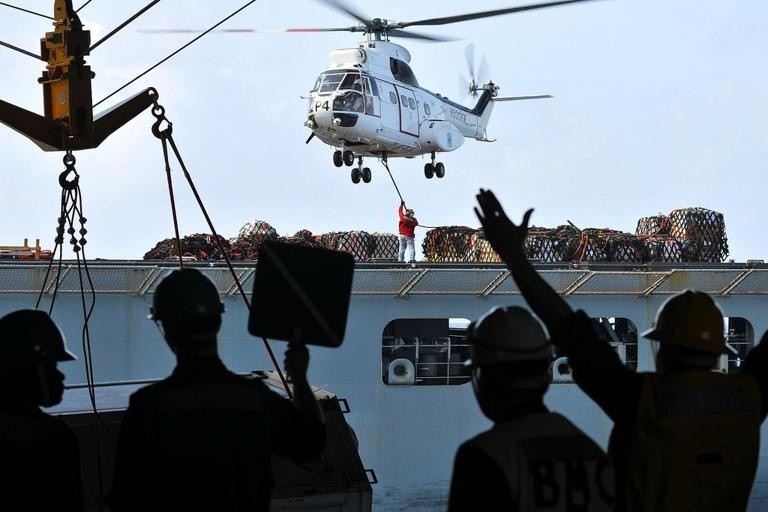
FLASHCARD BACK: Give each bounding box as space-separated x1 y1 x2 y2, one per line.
287 2 575 183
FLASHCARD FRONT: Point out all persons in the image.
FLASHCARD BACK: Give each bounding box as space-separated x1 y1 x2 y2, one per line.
116 267 326 511
447 303 610 512
474 186 768 512
398 200 418 263
345 74 365 111
1 308 79 511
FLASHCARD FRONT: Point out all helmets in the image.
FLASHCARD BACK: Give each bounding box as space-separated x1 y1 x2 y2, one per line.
406 208 414 215
641 289 739 357
0 309 76 364
468 307 553 360
147 266 226 322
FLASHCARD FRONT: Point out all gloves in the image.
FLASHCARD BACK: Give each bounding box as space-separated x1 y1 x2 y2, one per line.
401 200 406 205
